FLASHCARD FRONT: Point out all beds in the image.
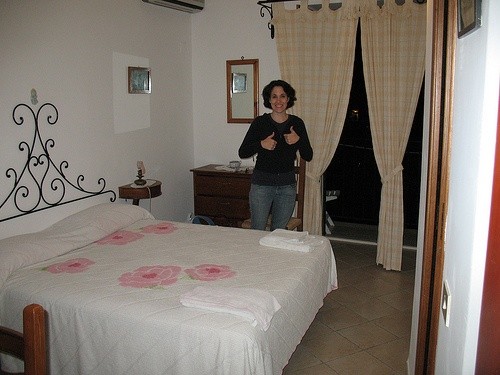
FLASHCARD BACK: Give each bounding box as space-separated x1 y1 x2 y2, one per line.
0 202 338 375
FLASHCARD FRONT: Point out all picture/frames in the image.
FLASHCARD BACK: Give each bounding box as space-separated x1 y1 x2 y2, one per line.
128 65 152 95
226 58 257 123
457 0 481 40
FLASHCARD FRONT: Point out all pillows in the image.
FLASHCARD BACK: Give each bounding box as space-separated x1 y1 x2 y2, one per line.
0 204 145 283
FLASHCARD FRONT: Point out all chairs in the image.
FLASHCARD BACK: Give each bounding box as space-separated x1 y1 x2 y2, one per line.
0 302 49 375
240 158 307 232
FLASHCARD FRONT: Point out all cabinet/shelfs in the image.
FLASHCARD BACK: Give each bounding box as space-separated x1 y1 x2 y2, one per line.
325 190 340 234
190 164 272 228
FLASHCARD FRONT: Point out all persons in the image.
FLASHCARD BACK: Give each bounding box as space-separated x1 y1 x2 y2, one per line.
238 80 314 231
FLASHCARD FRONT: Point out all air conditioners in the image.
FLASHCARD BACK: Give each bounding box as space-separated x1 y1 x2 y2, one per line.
143 0 205 15
